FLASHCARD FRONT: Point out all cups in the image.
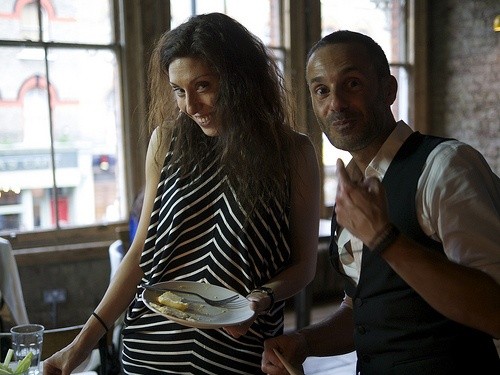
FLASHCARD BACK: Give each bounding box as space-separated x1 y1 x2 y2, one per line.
10 324 45 375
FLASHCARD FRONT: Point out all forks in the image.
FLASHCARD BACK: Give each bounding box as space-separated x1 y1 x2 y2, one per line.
142 283 239 306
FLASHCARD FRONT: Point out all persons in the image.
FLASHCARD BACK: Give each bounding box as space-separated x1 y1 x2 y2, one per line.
262 30 500 375
43 13 321 375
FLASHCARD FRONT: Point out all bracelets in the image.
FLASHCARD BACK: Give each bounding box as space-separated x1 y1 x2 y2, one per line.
92 311 109 333
366 223 402 258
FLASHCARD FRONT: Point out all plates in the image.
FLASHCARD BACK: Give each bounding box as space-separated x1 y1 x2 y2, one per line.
142 281 256 329
